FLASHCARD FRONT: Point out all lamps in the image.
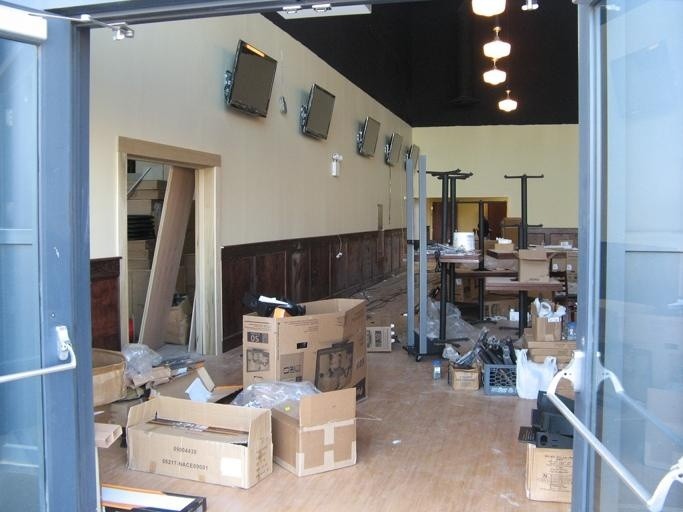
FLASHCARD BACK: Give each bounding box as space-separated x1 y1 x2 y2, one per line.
332 152 344 177
471 0 519 113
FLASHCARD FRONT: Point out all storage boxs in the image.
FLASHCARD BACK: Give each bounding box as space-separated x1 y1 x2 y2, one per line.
124 395 273 490
273 385 359 478
447 359 482 391
243 297 370 406
523 442 573 503
91 348 127 408
642 382 683 473
446 239 579 341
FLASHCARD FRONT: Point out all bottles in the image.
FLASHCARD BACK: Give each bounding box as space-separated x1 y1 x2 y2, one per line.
567 321 577 341
431 359 442 380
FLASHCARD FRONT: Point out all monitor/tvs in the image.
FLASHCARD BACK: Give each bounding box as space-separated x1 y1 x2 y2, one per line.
385 132 404 166
302 83 335 141
405 144 419 169
359 116 381 157
224 40 278 118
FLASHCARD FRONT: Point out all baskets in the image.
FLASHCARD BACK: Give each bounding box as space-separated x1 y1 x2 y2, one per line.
481 358 516 396
